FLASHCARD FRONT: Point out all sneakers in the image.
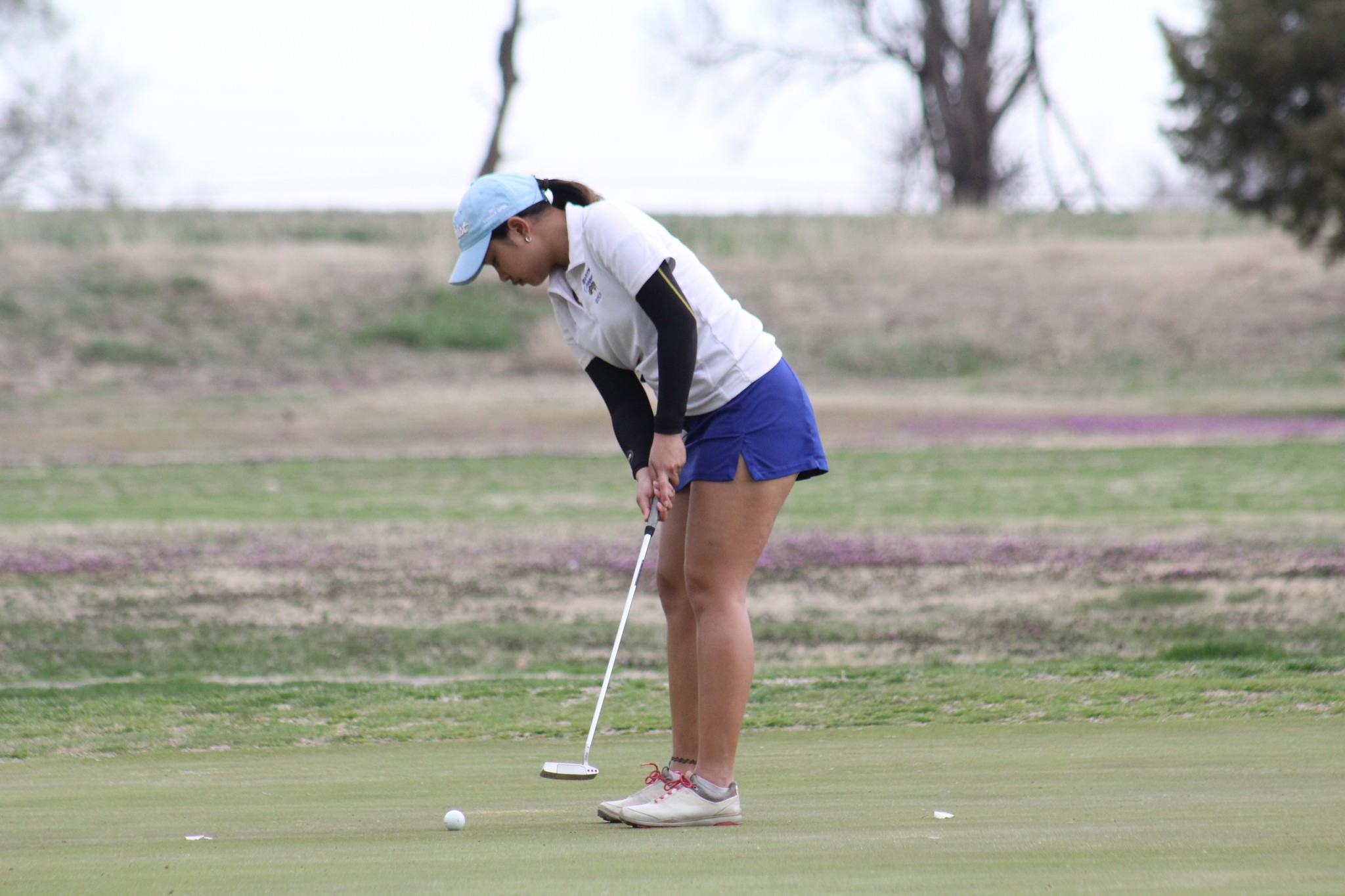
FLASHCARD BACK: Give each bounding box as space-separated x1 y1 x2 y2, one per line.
597 769 682 822
620 770 742 827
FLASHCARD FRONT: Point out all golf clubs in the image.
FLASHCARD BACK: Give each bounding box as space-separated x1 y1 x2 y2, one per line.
537 491 661 780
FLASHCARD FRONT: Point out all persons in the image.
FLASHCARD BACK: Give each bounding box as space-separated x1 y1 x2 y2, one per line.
449 171 829 826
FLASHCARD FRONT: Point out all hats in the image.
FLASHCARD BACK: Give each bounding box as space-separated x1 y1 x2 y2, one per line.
448 172 548 286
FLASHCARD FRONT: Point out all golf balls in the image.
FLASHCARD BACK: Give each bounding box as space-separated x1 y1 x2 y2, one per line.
444 810 465 831
